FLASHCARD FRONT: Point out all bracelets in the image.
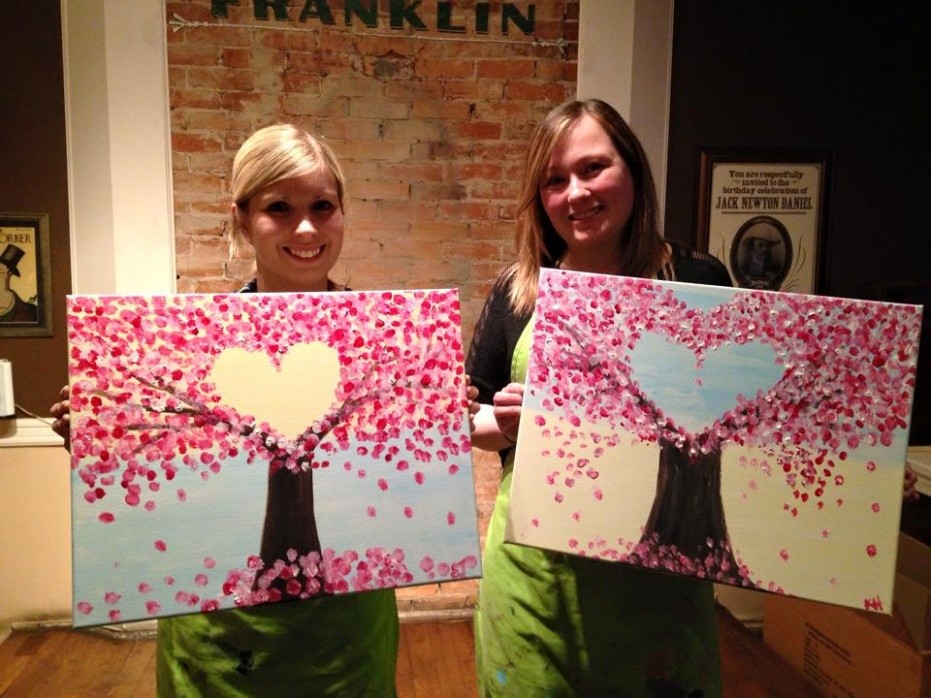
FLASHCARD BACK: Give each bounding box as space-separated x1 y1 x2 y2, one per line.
503 432 517 445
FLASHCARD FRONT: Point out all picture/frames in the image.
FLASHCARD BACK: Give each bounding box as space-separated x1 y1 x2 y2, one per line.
691 145 835 301
0 212 54 337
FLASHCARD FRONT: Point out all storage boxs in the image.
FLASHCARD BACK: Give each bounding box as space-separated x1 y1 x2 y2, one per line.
764 530 931 698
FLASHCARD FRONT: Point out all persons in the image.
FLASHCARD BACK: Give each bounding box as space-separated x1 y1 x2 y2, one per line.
466 99 919 698
50 121 480 698
